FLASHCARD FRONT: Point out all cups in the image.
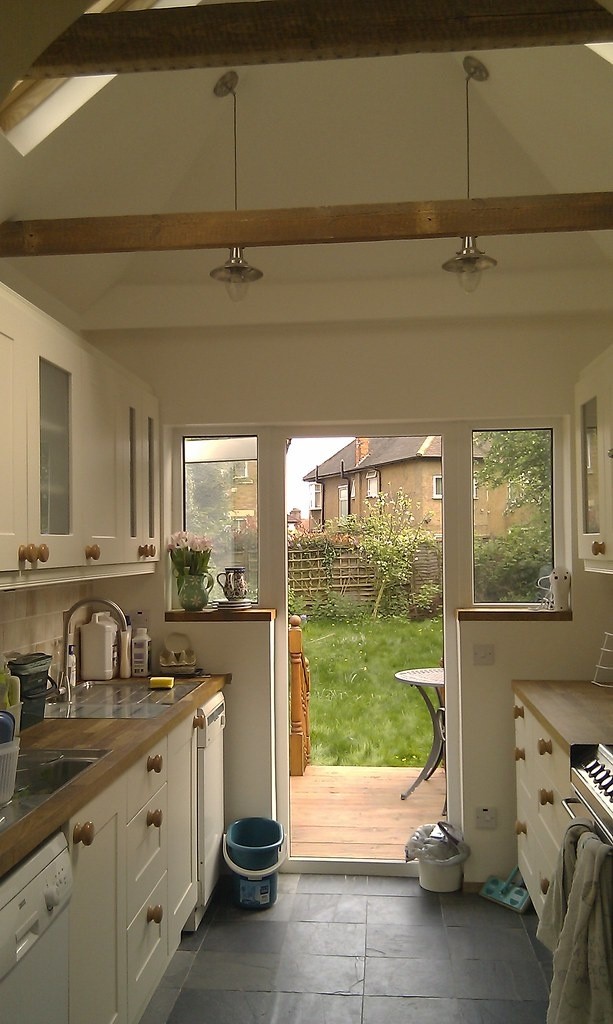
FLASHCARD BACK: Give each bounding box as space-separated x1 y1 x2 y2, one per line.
217 566 247 600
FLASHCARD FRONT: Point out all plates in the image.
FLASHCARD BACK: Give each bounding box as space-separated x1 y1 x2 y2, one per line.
212 599 252 609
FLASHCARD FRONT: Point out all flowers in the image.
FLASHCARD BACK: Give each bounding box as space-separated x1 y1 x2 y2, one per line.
171 531 213 578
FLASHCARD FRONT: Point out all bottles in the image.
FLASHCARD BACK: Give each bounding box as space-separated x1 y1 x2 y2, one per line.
551 561 570 611
68 645 76 686
120 615 132 678
132 628 152 677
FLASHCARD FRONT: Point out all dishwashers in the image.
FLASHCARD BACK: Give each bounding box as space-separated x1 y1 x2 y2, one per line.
198 691 225 906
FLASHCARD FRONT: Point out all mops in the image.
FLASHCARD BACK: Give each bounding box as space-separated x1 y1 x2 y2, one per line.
479 861 532 912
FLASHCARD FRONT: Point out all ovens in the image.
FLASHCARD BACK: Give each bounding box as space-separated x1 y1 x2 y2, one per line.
0 832 73 1024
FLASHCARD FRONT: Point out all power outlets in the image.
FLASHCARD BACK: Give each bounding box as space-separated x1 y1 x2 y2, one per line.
476 806 497 832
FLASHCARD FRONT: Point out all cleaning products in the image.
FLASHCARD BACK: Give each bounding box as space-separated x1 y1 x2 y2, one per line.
65 644 77 687
120 613 132 679
132 627 153 678
79 610 119 681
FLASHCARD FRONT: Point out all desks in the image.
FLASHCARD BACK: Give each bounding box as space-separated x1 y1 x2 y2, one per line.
395 665 447 819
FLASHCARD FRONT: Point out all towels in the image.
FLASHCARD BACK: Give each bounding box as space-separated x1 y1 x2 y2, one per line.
545 831 612 1024
536 817 596 954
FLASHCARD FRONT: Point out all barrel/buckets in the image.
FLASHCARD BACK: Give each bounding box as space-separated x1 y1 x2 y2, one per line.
222 816 288 909
81 611 120 680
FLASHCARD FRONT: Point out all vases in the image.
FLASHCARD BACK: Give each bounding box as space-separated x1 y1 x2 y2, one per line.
181 575 207 610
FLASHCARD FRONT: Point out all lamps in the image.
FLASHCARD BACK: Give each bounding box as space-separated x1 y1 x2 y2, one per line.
202 66 264 303
441 54 496 294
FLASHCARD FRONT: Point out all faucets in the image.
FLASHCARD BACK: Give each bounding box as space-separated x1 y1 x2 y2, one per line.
60 596 128 701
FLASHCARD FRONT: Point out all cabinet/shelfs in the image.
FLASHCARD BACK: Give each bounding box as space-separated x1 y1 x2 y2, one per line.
65 722 210 1024
514 690 587 919
0 281 161 595
573 352 613 575
